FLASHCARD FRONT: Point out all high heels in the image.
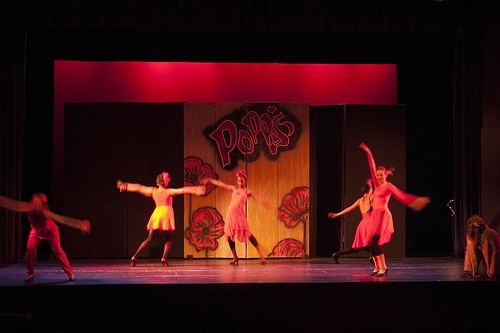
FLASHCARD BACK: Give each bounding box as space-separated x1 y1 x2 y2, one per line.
371 271 377 275
230 257 239 266
70 278 75 281
161 259 169 266
375 267 388 276
369 257 374 266
131 258 137 267
331 253 340 264
23 275 35 282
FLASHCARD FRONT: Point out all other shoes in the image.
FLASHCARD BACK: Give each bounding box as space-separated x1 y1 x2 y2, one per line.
464 271 473 278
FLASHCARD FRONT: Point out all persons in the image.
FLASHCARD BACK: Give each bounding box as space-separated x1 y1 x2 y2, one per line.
203 168 268 265
328 144 431 277
117 173 206 266
0 193 91 282
455 216 500 281
